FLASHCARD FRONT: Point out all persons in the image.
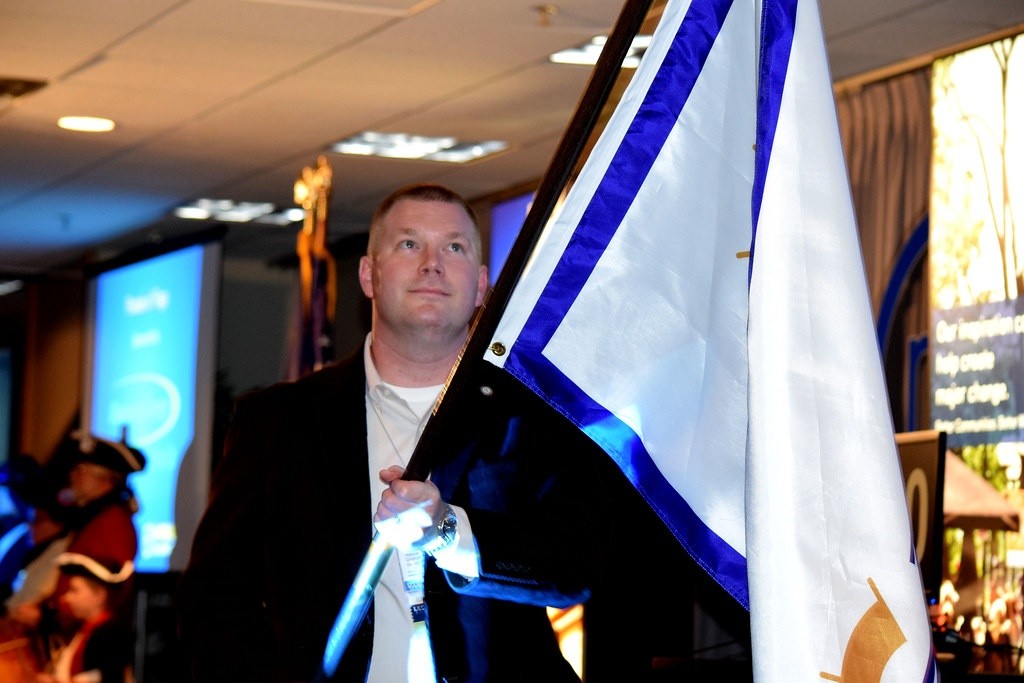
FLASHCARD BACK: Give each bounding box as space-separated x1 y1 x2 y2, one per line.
2 434 149 683
181 186 632 683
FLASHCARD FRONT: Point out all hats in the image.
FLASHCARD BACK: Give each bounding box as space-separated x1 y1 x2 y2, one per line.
63 429 146 473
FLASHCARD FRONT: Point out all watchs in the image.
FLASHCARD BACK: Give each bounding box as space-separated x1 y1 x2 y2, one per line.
417 504 461 557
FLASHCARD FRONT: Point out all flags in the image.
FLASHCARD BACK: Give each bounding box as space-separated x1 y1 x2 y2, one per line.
479 0 941 682
